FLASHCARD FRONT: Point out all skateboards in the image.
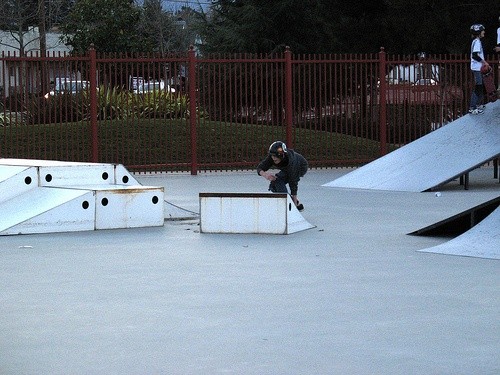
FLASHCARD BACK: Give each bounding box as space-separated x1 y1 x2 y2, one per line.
480 65 498 99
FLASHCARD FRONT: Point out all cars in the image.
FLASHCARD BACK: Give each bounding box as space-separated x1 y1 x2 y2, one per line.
138 82 175 94
45 81 101 103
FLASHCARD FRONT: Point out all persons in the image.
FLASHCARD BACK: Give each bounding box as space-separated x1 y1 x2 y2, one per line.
179 76 188 92
257 141 308 210
493 17 500 53
468 24 494 115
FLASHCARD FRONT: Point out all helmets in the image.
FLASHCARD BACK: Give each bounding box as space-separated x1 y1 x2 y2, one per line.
268 141 288 159
470 24 485 37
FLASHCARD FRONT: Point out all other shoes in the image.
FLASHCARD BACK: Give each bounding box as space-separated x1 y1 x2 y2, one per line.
476 105 486 109
469 108 484 116
